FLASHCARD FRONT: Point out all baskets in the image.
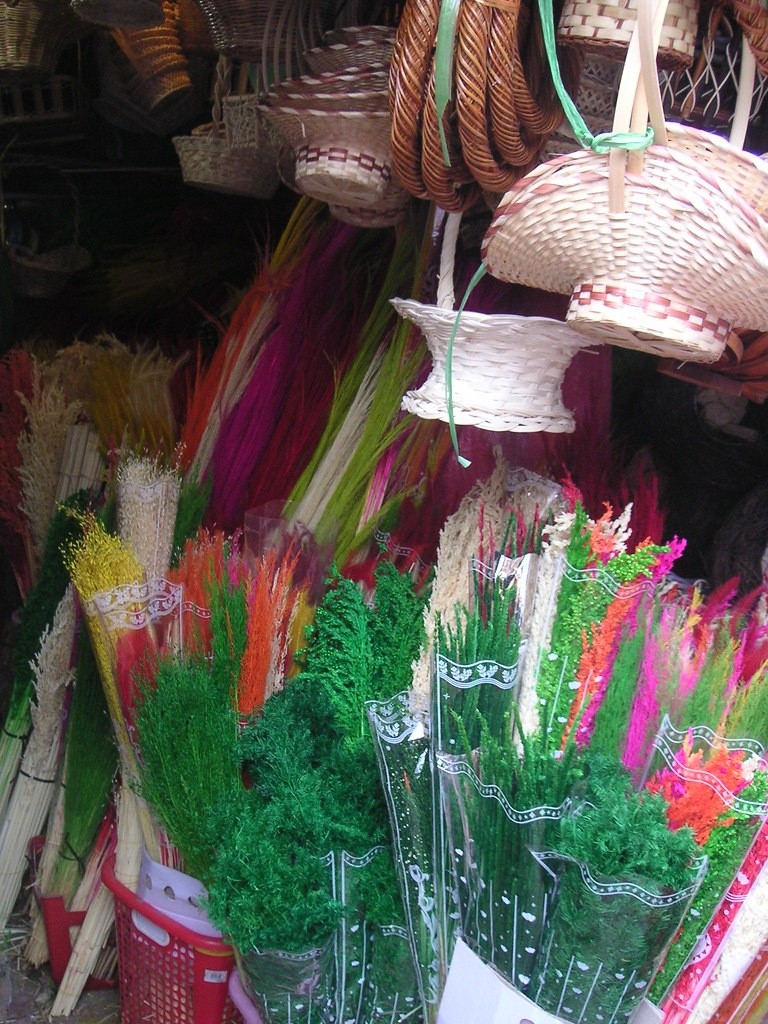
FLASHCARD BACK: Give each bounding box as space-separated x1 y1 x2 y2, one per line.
101 852 262 1024
0 0 768 433
